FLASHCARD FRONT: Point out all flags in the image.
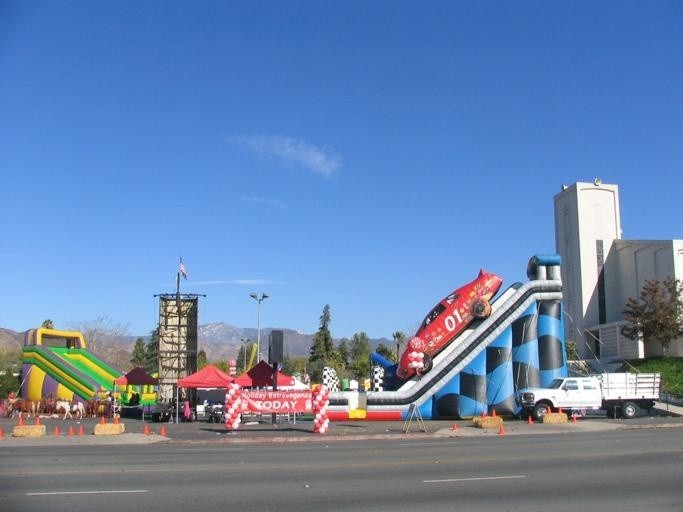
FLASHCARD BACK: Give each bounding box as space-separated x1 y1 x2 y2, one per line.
178 257 187 280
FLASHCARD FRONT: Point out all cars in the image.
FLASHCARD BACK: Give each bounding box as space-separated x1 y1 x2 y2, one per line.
395 267 504 381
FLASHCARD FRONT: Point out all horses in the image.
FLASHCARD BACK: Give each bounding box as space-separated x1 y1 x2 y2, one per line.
6 399 42 418
55 400 86 420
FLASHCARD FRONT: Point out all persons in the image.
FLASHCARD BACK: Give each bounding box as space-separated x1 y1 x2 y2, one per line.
104 390 113 417
183 397 191 422
6 390 18 412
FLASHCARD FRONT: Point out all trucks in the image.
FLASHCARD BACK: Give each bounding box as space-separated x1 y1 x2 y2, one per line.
513 373 661 420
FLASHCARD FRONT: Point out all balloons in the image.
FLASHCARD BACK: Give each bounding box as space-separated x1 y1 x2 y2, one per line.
222 383 241 431
407 336 426 374
311 384 331 434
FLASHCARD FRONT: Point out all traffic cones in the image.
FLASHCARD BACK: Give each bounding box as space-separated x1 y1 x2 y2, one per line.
157 424 168 436
52 424 86 436
99 415 107 425
143 425 152 435
452 406 577 436
113 416 120 425
1 426 5 439
17 415 40 427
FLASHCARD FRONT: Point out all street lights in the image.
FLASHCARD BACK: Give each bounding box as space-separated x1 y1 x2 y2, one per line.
248 290 269 369
240 336 250 371
